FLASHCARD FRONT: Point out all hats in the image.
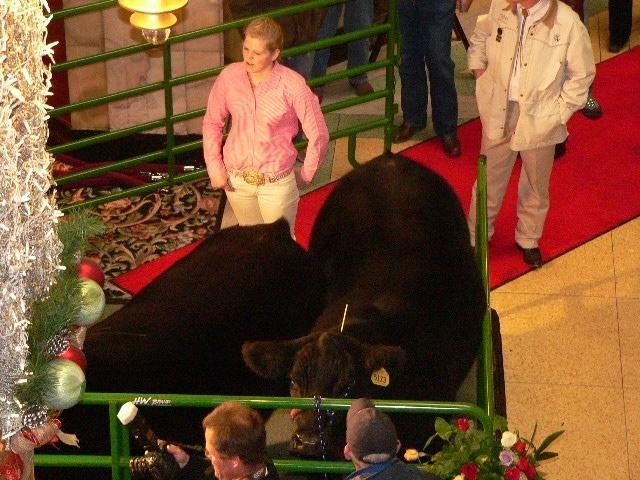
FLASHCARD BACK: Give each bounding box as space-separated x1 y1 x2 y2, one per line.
346 398 397 464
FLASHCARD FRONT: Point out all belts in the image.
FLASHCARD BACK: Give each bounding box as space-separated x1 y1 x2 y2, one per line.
232 169 293 187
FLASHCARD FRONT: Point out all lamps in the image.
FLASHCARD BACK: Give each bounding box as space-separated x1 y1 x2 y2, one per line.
117 1 191 46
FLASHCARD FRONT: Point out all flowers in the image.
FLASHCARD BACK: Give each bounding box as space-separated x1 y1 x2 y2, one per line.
405 414 565 480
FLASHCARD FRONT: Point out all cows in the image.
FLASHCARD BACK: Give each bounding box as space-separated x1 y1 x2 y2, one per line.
240 150 488 465
33 215 326 480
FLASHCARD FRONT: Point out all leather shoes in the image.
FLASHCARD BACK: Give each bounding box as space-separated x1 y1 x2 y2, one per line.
391 122 424 143
443 134 461 156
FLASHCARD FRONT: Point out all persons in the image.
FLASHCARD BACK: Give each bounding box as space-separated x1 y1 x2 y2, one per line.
157 401 279 480
392 1 465 157
340 398 440 480
561 0 633 118
201 16 330 242
465 0 596 269
227 0 374 103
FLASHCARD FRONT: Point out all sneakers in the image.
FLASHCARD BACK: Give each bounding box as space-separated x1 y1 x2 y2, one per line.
312 86 324 103
515 244 542 268
608 42 622 52
581 95 601 119
350 81 374 96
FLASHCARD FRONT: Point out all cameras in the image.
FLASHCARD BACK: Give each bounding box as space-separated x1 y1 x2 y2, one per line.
117 401 181 480
